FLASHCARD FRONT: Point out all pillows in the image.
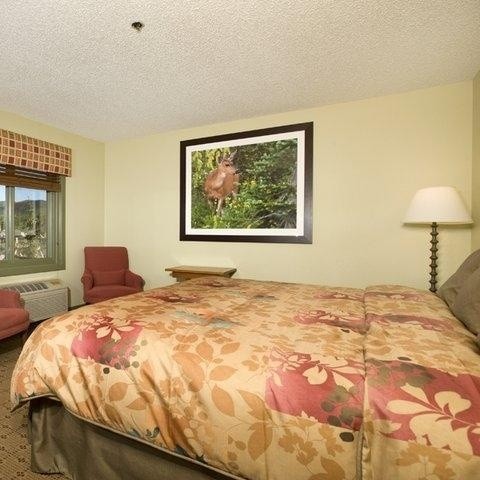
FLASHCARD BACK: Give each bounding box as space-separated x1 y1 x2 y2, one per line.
435 249 478 339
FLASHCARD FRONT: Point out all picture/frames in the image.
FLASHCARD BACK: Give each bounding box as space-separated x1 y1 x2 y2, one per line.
180 121 313 243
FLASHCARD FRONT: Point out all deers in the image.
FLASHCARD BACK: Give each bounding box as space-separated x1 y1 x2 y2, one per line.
203 149 239 215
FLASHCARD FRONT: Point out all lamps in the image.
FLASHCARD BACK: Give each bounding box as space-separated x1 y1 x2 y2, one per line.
403 187 473 293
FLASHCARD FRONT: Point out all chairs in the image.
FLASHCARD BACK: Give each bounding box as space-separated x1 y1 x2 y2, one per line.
0 289 30 346
81 246 144 303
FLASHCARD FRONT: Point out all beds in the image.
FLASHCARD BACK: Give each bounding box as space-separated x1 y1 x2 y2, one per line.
25 274 480 479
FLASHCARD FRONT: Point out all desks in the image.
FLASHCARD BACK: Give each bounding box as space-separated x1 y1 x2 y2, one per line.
165 265 236 282
